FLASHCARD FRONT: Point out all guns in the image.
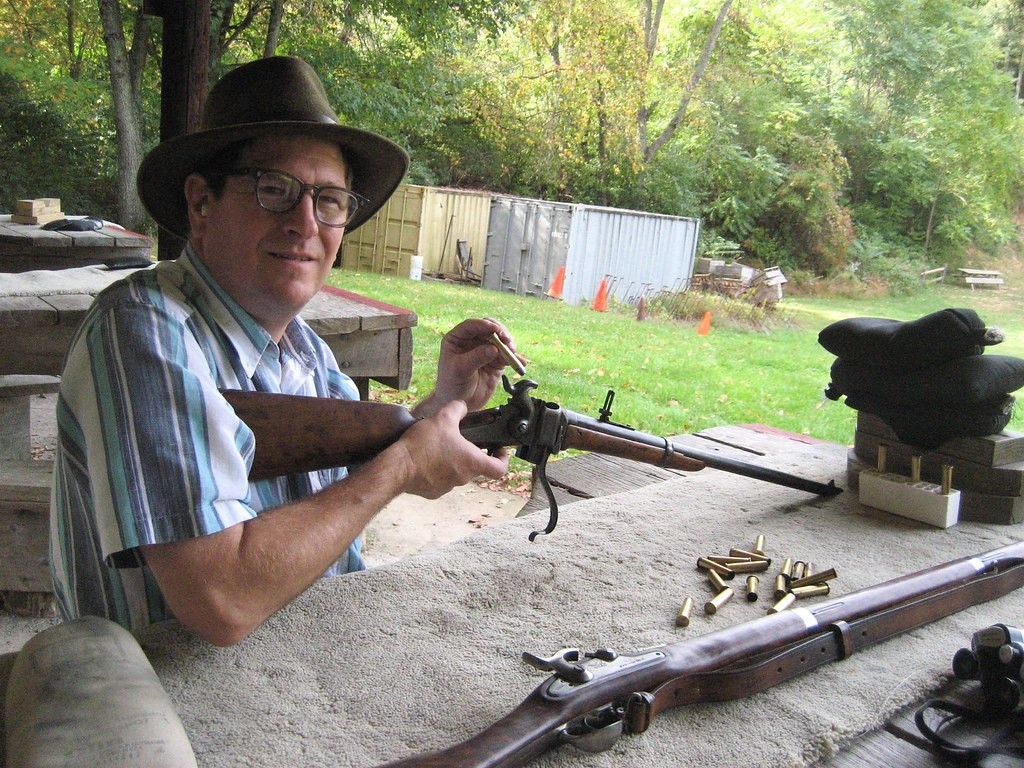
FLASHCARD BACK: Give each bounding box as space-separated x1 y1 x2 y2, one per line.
221 374 845 535
383 542 1024 768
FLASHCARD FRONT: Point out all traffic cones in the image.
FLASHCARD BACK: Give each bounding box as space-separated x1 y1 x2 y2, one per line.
589 278 609 313
699 312 710 334
637 296 646 321
543 267 565 300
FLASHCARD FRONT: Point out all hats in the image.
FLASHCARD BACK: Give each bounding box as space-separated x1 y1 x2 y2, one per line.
137 56 410 242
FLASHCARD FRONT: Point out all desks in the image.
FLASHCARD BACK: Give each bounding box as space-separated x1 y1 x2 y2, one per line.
0 423 1024 767
955 268 1001 292
0 259 418 618
0 214 154 273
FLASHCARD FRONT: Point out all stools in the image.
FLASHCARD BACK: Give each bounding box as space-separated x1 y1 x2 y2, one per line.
1 375 61 461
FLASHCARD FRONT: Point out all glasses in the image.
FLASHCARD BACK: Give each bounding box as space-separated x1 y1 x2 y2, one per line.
226 166 369 228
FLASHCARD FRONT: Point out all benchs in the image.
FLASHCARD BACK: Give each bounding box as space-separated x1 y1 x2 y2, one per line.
951 274 1003 284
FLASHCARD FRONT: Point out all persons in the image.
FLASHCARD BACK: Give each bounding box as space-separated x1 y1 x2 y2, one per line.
48 54 528 649
